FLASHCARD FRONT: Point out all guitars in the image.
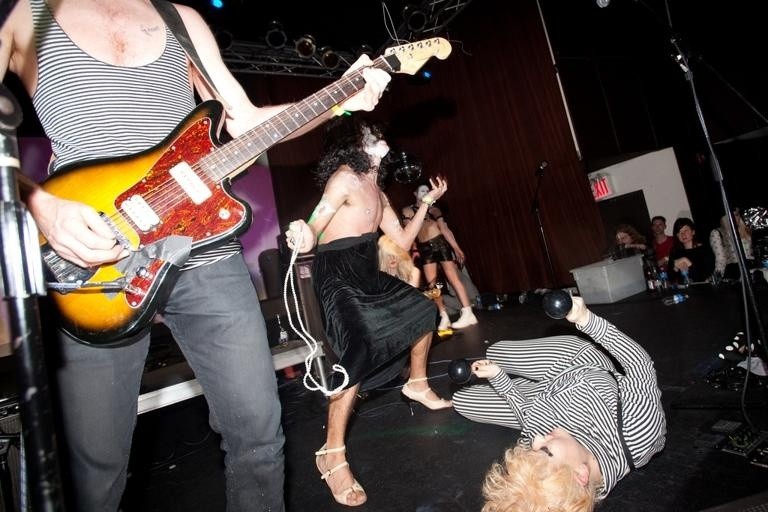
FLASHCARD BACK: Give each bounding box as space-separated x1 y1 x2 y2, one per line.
27 36 451 349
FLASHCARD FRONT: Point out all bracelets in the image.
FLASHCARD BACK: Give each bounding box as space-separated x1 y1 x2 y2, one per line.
331 105 344 117
422 196 436 207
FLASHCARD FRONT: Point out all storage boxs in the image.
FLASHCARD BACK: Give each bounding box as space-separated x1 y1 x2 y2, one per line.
569 253 645 305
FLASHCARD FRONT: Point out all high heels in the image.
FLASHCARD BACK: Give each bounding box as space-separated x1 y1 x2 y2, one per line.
315 443 367 506
401 377 452 416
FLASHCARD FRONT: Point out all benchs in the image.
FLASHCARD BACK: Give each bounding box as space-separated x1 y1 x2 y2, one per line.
137 338 326 419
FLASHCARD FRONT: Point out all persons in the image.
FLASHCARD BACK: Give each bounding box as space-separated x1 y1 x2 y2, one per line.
615 203 768 285
0 0 393 512
452 297 667 512
378 235 421 287
285 111 453 507
402 184 479 330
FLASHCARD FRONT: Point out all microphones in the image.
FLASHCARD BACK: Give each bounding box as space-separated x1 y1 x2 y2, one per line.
534 158 548 177
596 0 611 9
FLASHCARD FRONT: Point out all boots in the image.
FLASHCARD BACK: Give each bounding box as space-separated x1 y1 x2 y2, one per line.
438 307 477 331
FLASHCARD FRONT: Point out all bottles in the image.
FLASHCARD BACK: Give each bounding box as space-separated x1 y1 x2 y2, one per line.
646 266 670 292
663 294 690 306
275 314 289 348
681 271 691 285
